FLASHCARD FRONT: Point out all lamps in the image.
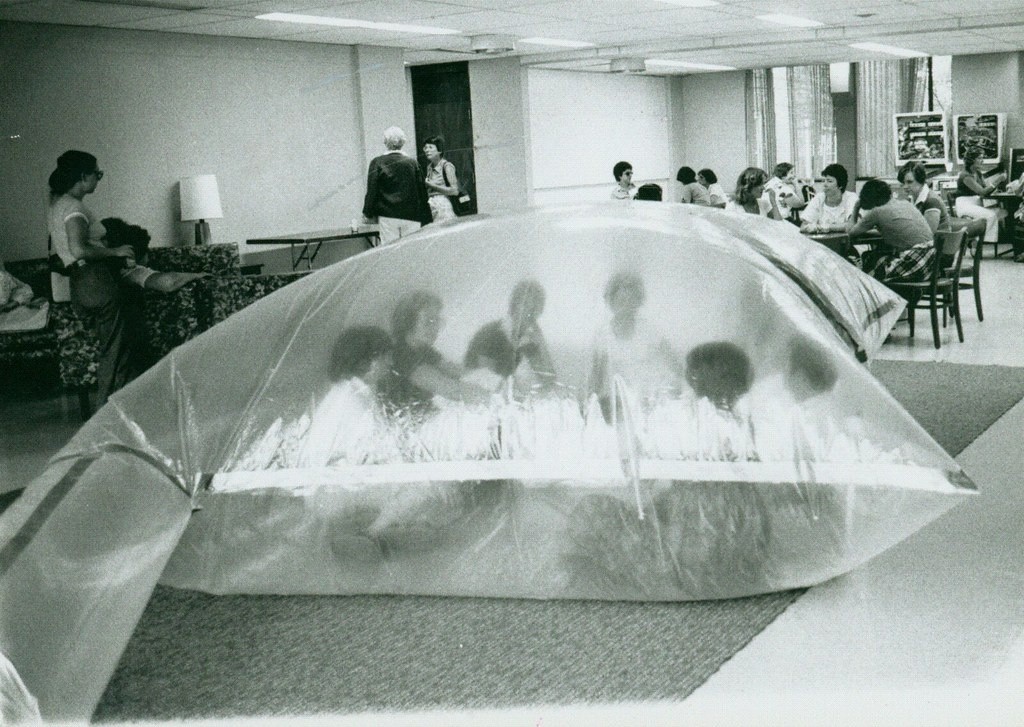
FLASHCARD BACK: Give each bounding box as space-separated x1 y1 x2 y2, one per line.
179 174 224 244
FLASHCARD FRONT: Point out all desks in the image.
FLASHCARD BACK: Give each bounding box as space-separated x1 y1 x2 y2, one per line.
984 192 1024 261
807 232 886 273
246 226 381 272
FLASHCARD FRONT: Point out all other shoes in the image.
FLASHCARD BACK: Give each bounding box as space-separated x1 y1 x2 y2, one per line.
967 242 976 257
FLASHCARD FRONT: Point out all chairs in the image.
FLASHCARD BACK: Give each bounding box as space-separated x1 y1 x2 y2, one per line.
883 191 1001 349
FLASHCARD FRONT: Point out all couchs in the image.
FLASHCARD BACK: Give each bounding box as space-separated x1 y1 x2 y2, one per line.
0 242 301 396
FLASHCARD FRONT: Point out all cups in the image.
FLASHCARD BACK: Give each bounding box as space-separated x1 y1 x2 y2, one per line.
351 219 359 232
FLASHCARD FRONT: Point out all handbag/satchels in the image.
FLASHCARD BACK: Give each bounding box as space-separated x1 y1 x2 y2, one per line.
442 162 472 215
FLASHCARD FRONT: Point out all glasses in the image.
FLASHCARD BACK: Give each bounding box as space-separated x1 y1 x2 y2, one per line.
84 169 104 180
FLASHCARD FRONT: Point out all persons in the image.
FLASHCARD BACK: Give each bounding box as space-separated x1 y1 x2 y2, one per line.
846 180 936 305
610 161 640 199
725 167 783 221
0 270 34 314
897 161 954 267
46 150 136 411
677 166 711 206
764 162 804 220
423 136 458 222
955 147 1007 256
1013 173 1024 263
299 270 851 592
801 163 868 267
120 246 214 293
362 126 429 245
697 169 729 207
638 183 662 202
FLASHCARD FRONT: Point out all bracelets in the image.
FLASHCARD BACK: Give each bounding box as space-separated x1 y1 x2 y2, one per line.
991 184 995 188
